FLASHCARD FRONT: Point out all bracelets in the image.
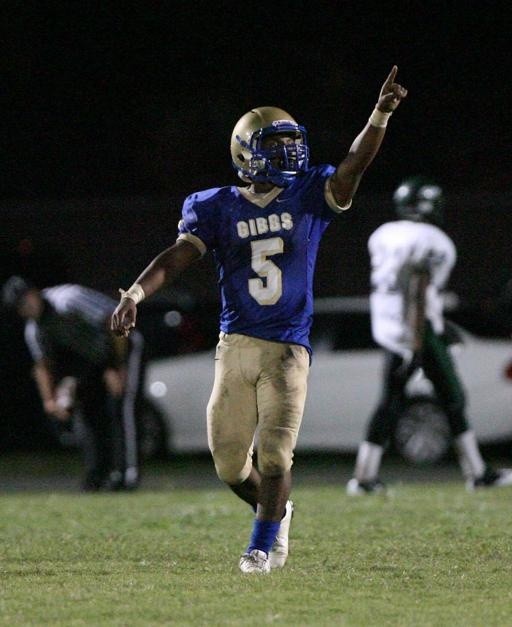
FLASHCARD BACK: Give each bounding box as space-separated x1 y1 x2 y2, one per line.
118 281 145 305
367 108 393 129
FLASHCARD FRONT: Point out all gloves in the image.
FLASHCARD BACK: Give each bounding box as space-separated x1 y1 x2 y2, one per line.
396 347 418 377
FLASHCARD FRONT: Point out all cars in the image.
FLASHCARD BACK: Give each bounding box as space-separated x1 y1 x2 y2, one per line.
54 296 512 468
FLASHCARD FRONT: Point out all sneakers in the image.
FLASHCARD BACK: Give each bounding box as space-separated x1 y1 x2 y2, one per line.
239 499 294 574
465 468 512 492
347 479 385 496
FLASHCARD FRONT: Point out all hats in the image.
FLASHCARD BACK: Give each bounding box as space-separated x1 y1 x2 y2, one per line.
4 274 28 307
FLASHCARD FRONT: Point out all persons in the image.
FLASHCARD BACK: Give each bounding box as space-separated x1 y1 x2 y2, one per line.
112 63 407 566
346 171 512 496
1 276 149 491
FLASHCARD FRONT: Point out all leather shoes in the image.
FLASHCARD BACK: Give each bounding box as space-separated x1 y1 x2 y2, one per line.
80 466 141 492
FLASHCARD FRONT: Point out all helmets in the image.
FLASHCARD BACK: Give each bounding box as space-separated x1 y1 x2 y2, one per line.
230 106 311 178
394 177 444 216
